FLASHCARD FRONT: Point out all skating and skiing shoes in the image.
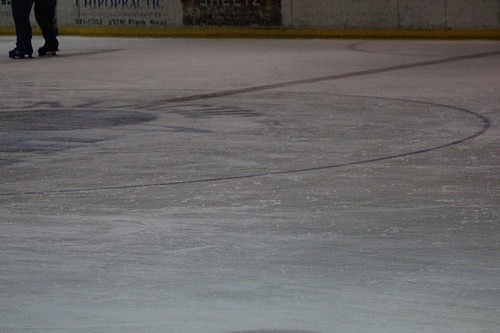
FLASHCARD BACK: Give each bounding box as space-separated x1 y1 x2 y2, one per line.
9 41 32 59
37 39 59 56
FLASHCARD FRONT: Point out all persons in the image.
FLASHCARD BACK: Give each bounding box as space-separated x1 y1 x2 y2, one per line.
8 0 59 59
